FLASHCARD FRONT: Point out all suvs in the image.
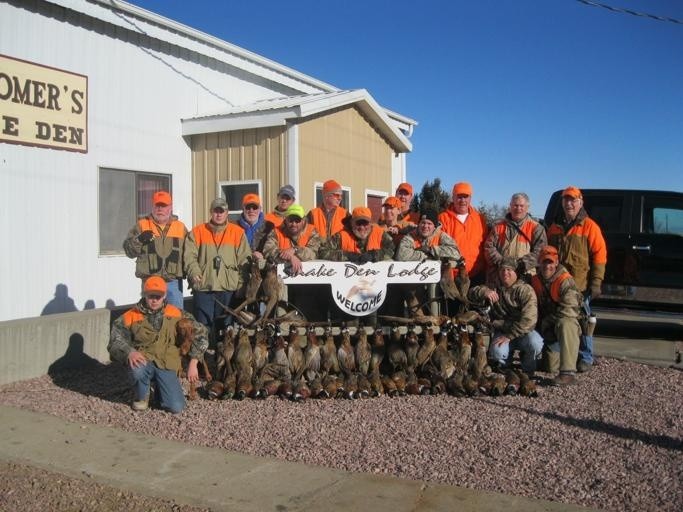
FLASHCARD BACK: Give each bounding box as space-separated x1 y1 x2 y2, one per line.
544 189 682 336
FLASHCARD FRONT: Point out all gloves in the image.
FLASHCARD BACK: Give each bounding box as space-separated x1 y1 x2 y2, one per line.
345 251 367 264
414 245 432 256
365 249 385 262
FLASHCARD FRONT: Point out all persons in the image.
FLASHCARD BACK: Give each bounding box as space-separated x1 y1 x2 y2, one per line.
526 244 584 387
106 275 209 414
125 179 461 377
436 181 488 287
483 192 549 281
467 257 543 384
546 184 609 373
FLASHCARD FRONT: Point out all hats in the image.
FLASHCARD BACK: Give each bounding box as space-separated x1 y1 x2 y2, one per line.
561 187 580 200
284 203 305 218
381 196 402 208
396 183 413 195
453 182 471 196
321 181 342 192
278 185 295 198
210 198 228 212
539 246 558 264
242 193 260 207
154 191 172 207
419 210 438 227
501 257 519 271
352 206 371 222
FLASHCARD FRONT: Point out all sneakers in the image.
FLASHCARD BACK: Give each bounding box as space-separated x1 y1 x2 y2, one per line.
551 374 575 386
577 360 592 371
132 386 150 411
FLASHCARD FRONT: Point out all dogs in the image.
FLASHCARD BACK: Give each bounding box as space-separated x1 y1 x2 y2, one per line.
174 319 211 401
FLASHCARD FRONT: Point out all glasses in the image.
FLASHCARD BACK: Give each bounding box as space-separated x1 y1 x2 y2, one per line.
354 219 370 226
245 204 258 211
288 215 302 224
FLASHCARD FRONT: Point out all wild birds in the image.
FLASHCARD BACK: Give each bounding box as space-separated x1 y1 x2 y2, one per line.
205 255 538 401
